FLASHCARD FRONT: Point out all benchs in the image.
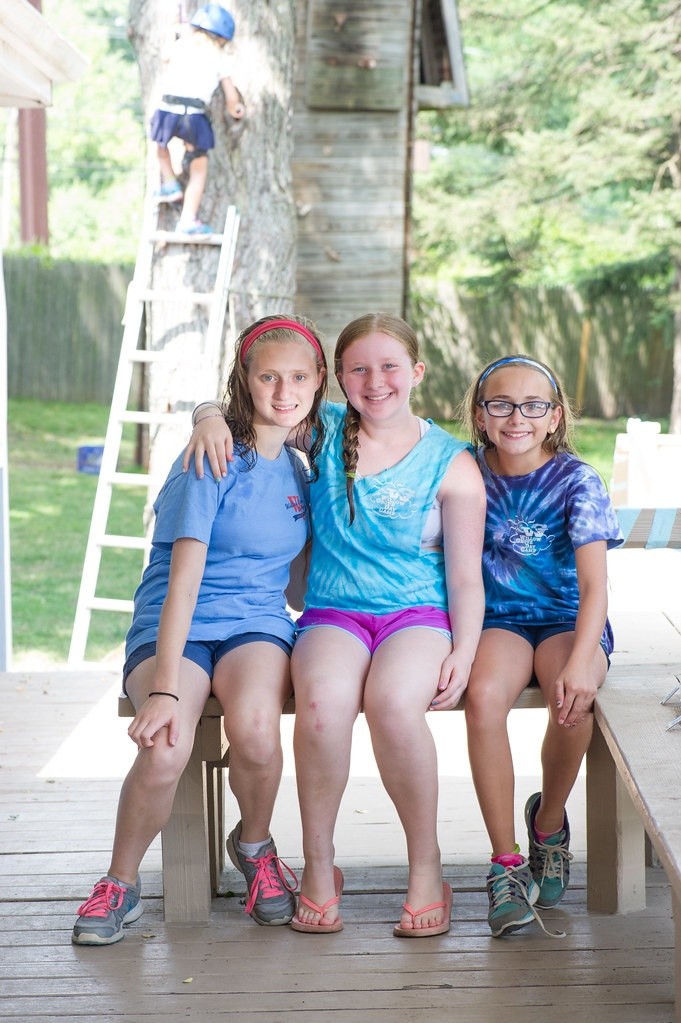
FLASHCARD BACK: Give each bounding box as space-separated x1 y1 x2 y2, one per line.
118 664 681 1022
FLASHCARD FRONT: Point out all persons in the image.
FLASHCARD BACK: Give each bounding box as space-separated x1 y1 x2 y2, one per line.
70 313 328 946
181 311 486 936
464 356 625 938
147 4 246 235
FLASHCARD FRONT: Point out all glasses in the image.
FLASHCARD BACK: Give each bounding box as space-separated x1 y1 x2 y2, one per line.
478 400 555 418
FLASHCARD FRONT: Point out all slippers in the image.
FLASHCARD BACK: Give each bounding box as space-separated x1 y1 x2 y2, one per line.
394 881 453 937
291 865 344 933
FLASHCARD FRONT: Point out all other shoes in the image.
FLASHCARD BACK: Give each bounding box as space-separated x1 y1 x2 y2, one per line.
176 221 215 237
157 182 184 203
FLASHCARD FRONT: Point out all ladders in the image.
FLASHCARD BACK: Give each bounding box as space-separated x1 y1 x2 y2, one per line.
66 199 243 670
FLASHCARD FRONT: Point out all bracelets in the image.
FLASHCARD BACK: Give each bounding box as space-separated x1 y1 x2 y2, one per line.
191 402 225 427
148 691 179 702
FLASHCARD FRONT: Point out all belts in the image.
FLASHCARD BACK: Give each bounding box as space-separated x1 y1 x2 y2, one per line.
158 101 206 117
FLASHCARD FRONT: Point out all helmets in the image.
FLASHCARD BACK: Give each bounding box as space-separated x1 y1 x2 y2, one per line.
190 5 235 41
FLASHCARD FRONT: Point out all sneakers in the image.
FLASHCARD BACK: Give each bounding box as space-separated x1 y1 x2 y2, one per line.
486 854 567 939
72 873 144 946
524 792 573 909
227 820 298 926
